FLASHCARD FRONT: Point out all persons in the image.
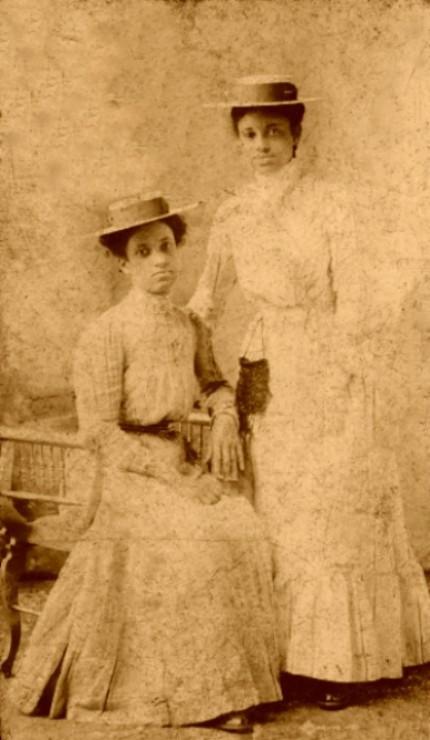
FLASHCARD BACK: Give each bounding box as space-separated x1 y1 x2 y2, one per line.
183 74 430 711
8 190 268 733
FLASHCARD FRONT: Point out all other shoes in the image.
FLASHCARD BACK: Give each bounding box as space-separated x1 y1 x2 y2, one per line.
314 684 348 709
211 712 255 733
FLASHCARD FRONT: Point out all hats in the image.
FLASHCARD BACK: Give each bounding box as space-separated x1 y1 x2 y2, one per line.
198 73 314 108
90 188 202 239
